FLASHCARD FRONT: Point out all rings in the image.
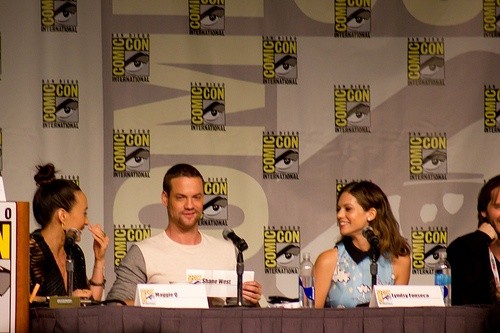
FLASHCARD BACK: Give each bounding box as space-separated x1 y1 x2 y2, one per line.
103 231 106 238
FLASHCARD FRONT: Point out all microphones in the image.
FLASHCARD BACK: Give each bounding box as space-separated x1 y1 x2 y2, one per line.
222 228 248 252
362 226 379 246
64 227 78 251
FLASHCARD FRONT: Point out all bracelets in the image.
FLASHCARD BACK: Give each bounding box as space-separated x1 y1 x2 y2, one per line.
89 278 106 286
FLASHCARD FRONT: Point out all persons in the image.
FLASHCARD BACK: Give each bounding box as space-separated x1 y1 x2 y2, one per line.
313 180 411 308
447 176 500 306
106 164 263 306
30 162 110 306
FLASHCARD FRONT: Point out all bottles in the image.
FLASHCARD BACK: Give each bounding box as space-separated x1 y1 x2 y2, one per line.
299 255 315 308
434 252 451 307
